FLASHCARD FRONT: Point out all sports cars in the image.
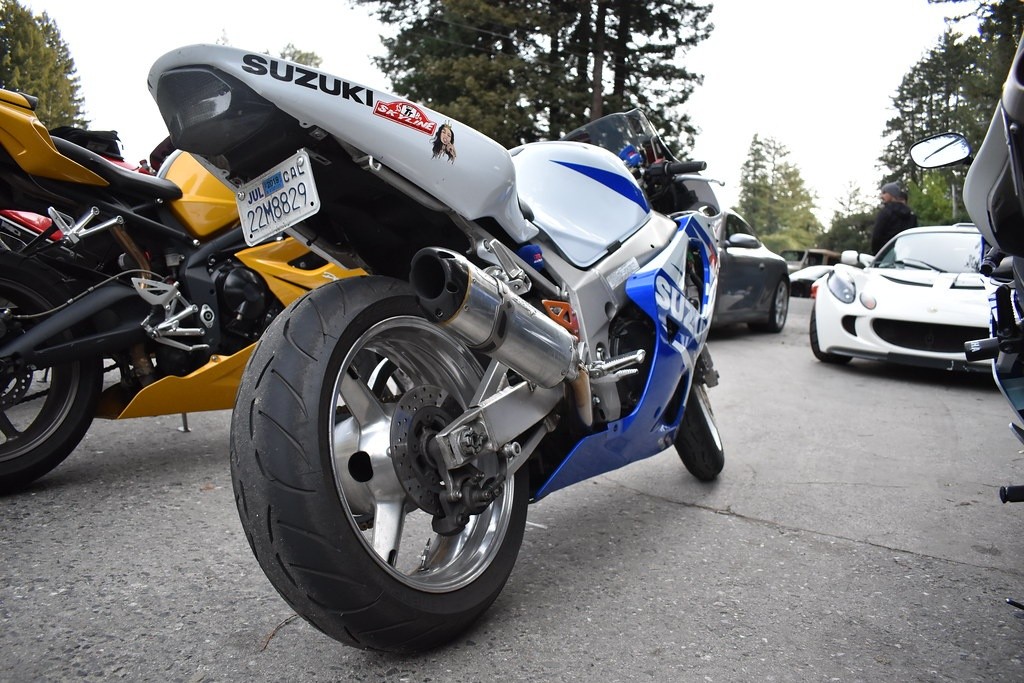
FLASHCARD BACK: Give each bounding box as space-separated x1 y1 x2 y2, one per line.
807 221 996 375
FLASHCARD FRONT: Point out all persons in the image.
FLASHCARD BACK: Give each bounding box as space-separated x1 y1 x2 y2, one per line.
431 124 456 164
871 182 917 255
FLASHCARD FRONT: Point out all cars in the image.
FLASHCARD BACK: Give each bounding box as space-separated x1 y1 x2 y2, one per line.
789 265 837 297
781 247 841 274
712 204 793 335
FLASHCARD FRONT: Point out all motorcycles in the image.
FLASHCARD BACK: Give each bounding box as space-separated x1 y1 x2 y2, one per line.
1 84 374 496
144 43 724 658
907 28 1024 513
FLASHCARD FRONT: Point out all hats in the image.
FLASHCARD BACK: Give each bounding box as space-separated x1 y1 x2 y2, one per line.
883 182 901 197
